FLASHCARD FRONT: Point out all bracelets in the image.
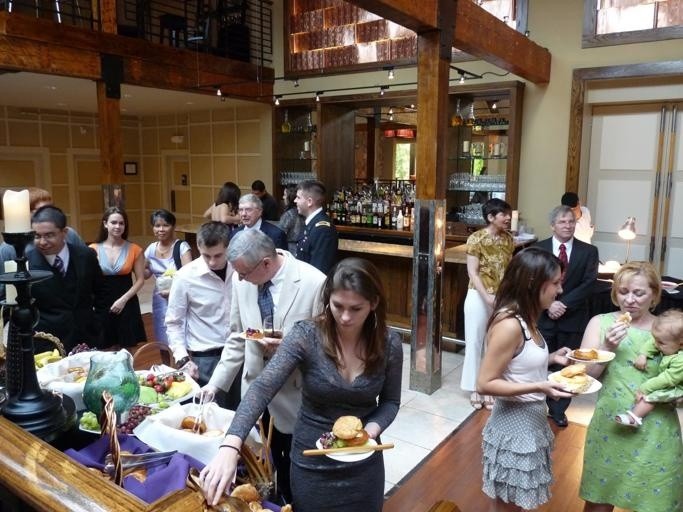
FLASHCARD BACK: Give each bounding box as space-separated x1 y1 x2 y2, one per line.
217 445 245 456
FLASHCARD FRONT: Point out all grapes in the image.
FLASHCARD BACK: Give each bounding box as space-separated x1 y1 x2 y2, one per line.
320 432 336 449
115 405 151 434
72 343 91 355
79 412 101 433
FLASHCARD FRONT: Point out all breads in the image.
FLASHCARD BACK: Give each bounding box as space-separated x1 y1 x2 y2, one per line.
346 431 368 448
574 348 598 359
182 416 206 432
562 363 587 377
333 415 363 440
231 484 260 504
557 376 590 386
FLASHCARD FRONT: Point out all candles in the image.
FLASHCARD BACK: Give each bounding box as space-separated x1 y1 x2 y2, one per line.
5 259 29 302
2 188 32 233
512 209 518 218
511 218 518 232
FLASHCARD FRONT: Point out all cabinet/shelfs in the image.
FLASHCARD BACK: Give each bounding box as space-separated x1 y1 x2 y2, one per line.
446 79 525 235
274 98 356 210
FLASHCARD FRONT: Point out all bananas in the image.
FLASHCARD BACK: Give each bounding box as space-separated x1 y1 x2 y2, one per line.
34 348 61 367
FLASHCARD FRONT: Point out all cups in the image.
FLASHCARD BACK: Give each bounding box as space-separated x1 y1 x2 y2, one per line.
450 174 506 191
461 203 485 225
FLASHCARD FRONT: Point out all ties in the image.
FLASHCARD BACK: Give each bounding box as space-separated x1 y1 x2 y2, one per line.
255 279 277 368
51 253 62 282
557 244 571 302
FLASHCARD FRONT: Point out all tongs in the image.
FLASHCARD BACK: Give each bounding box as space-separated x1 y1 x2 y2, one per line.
192 389 215 436
104 449 179 473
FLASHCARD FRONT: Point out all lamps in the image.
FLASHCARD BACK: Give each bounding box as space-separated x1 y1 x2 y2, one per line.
383 66 394 79
380 86 390 96
618 216 636 262
274 95 282 106
315 91 323 101
458 70 465 84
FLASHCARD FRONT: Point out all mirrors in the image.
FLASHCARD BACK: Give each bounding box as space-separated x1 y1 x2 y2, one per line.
353 104 416 191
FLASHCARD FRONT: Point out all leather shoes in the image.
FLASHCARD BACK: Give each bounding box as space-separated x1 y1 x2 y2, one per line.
551 413 568 429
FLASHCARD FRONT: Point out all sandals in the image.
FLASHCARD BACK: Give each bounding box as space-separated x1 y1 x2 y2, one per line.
613 409 645 430
483 394 495 411
470 392 483 410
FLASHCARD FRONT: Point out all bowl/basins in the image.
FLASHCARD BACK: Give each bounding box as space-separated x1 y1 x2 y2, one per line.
565 348 616 367
238 330 283 344
315 438 378 463
547 371 604 396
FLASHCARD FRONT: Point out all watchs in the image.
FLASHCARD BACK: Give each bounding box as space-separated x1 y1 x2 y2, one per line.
174 356 192 370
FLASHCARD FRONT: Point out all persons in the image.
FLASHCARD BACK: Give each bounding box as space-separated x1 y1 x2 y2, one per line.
194 227 326 512
88 205 147 354
24 186 90 270
459 196 517 411
16 204 110 351
251 178 280 228
559 190 594 244
202 180 242 232
226 192 290 253
477 248 579 512
164 220 243 409
293 179 339 278
584 259 683 512
132 207 195 370
0 228 17 365
525 204 600 428
275 182 304 258
617 309 683 431
194 256 405 512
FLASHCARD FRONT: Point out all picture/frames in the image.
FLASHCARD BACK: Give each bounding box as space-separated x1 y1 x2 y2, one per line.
124 162 137 174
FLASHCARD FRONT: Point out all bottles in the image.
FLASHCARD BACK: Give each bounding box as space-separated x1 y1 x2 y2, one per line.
377 203 383 228
403 206 409 231
384 200 392 228
350 205 356 225
307 111 313 135
356 203 361 225
366 201 371 226
325 202 330 218
281 109 291 132
342 201 346 224
361 201 366 226
337 200 342 224
466 101 475 126
331 202 337 224
372 203 377 227
397 209 403 230
345 201 351 225
392 207 396 229
452 97 463 127
